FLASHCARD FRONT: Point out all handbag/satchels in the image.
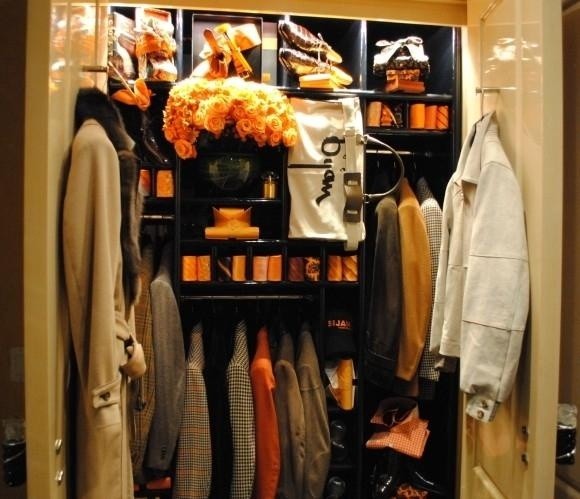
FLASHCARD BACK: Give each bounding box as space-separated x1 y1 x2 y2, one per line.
286 96 405 251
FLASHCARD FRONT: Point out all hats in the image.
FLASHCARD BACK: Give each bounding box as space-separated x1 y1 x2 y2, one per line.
323 305 358 359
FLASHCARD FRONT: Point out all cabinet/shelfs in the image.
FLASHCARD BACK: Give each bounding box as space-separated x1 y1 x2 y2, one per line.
107 6 460 499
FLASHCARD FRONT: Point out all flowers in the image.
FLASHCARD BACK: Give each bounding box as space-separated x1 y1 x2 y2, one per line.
160 70 299 163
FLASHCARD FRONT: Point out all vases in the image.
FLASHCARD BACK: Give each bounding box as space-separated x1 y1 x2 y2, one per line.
201 134 257 200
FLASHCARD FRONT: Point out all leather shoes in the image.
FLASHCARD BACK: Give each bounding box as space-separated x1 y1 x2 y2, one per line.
278 47 354 86
371 453 404 499
278 20 344 67
403 458 446 495
